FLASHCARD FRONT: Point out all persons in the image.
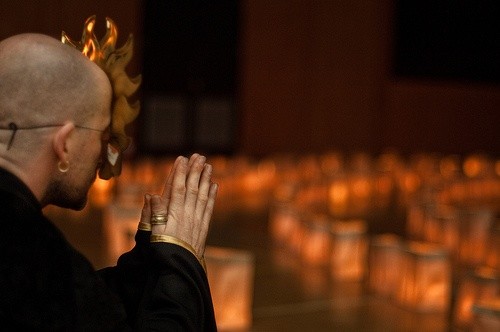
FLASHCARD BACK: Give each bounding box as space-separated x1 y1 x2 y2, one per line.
0 32 218 332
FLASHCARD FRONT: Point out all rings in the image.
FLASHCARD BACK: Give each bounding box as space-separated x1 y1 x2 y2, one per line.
150 213 168 225
